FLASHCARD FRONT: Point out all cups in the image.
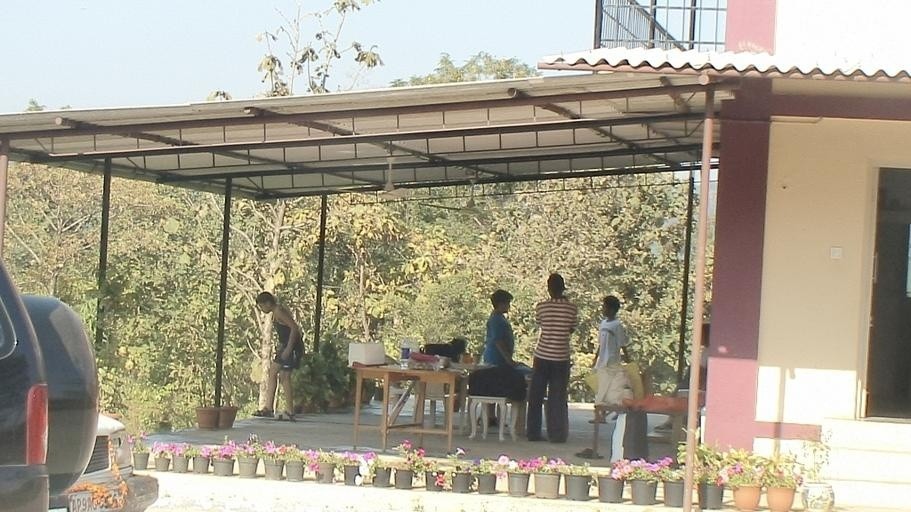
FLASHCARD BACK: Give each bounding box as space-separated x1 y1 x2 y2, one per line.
472 353 482 364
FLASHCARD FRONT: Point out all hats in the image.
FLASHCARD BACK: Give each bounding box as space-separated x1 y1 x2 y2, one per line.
546 273 569 290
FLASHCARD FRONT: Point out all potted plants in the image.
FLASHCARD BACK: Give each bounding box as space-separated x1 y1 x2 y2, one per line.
189 366 220 431
213 370 241 429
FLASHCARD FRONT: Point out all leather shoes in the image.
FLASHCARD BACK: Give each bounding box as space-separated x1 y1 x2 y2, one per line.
528 431 566 443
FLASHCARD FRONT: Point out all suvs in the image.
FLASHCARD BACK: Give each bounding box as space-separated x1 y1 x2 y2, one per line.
1 257 108 511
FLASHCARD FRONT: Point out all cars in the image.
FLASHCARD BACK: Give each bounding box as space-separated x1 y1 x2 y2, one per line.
48 413 136 511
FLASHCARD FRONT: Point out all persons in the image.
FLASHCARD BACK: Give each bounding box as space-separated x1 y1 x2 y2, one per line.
248 291 305 423
478 289 532 426
585 295 632 424
654 322 711 432
525 273 578 444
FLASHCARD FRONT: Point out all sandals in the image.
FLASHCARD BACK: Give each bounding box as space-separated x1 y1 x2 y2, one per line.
271 411 298 422
253 407 273 417
656 422 674 432
590 415 606 424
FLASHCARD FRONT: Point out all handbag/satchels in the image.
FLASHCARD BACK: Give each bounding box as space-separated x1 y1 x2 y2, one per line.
270 320 303 368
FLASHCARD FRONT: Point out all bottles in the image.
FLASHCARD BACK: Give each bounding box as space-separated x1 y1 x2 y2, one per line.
400 341 410 369
